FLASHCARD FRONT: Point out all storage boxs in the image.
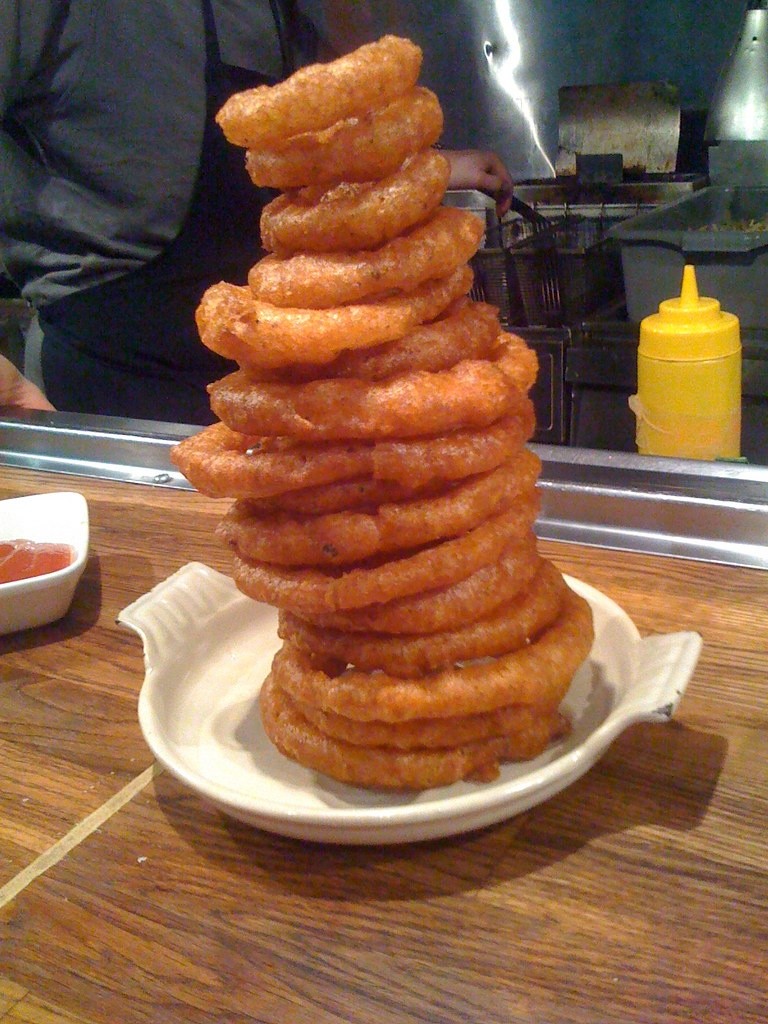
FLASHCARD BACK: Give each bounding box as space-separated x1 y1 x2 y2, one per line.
609 183 768 331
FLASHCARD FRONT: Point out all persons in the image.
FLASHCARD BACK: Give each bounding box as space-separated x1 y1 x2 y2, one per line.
0 0 514 426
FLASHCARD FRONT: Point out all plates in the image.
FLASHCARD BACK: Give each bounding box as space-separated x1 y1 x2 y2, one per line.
117 563 703 847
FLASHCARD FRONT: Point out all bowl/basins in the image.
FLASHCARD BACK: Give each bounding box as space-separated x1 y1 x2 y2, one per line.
0 492 90 635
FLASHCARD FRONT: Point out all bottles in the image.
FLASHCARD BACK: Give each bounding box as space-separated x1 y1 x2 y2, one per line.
636 265 743 459
